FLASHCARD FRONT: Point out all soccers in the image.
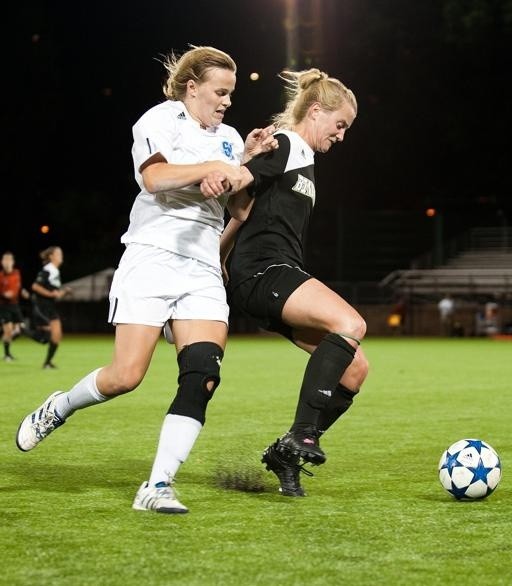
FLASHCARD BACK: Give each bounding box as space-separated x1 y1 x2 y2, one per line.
438 438 503 500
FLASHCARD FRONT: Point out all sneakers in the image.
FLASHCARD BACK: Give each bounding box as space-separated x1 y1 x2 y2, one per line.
133 481 190 514
262 438 306 498
277 428 326 465
17 391 71 450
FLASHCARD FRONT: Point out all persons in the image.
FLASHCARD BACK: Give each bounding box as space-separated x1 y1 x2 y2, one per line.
435 293 454 337
470 296 487 338
199 63 371 504
388 296 407 335
9 243 74 371
14 46 281 517
0 249 38 361
483 294 501 335
450 320 465 337
